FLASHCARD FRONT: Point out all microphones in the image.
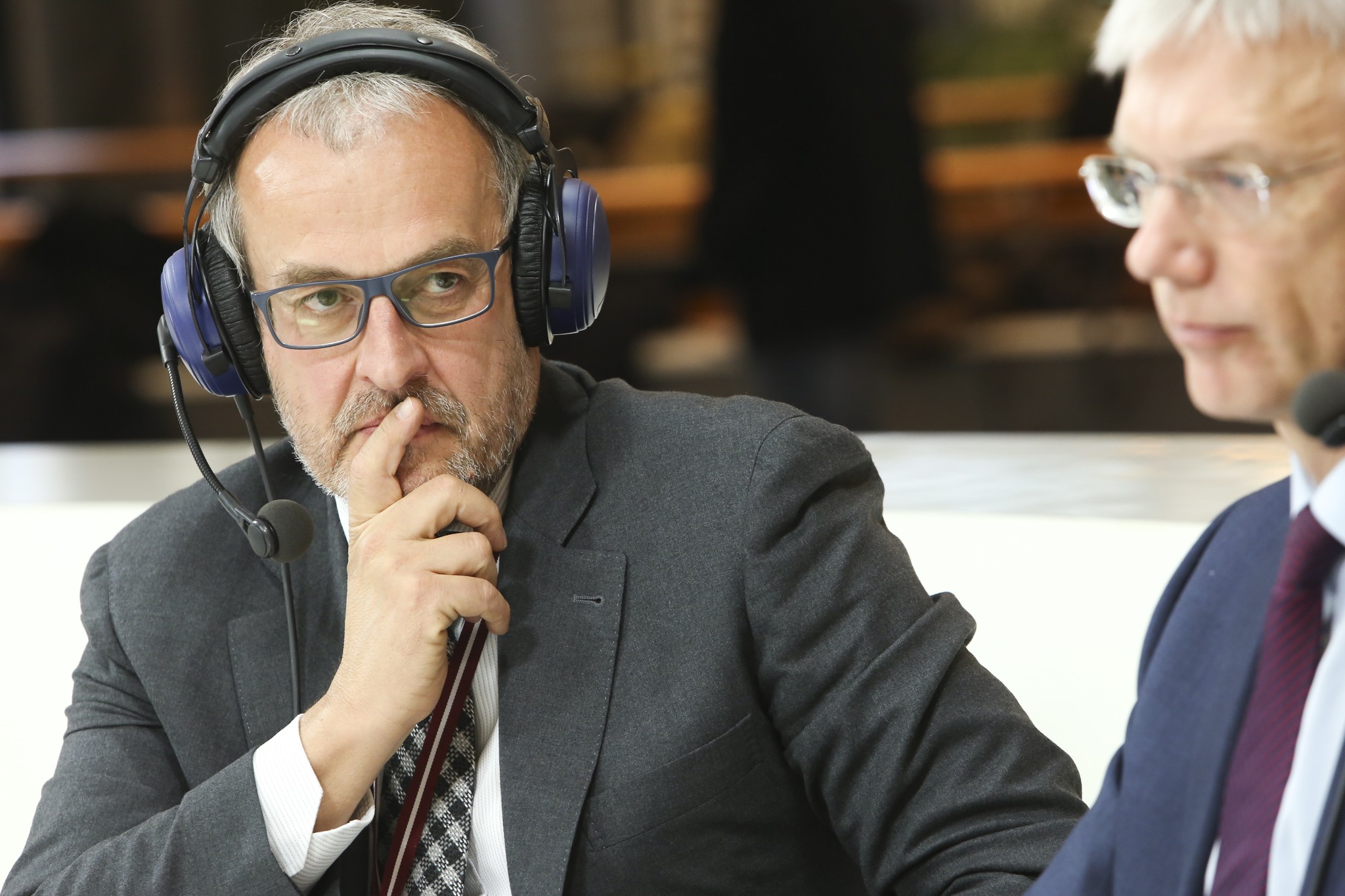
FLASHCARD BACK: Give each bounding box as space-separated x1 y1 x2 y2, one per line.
1294 368 1345 447
153 317 315 563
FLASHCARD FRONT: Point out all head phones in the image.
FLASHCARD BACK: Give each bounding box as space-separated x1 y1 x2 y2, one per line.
152 23 614 402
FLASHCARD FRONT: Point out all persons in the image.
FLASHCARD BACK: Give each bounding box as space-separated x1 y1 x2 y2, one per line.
0 3 1089 894
1014 0 1345 896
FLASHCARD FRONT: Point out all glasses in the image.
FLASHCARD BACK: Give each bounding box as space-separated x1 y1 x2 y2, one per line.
250 225 515 349
1081 146 1343 232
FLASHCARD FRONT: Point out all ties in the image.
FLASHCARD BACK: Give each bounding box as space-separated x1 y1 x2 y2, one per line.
1209 508 1343 896
372 638 477 894
